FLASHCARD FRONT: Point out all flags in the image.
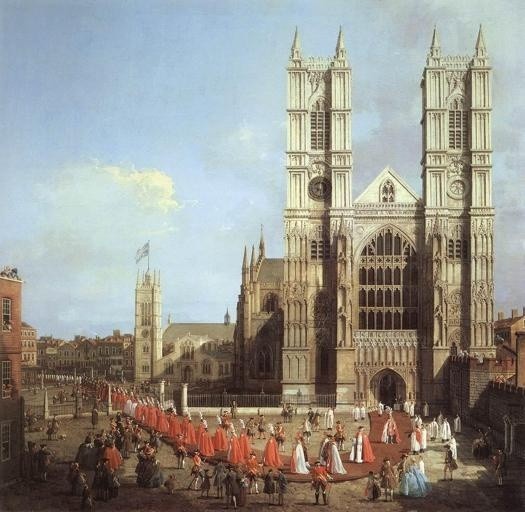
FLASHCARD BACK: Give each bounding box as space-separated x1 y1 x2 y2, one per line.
134 241 149 263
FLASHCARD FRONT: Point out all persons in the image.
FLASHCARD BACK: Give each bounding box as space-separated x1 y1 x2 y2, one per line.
494 447 506 487
19 388 465 512
471 424 496 456
